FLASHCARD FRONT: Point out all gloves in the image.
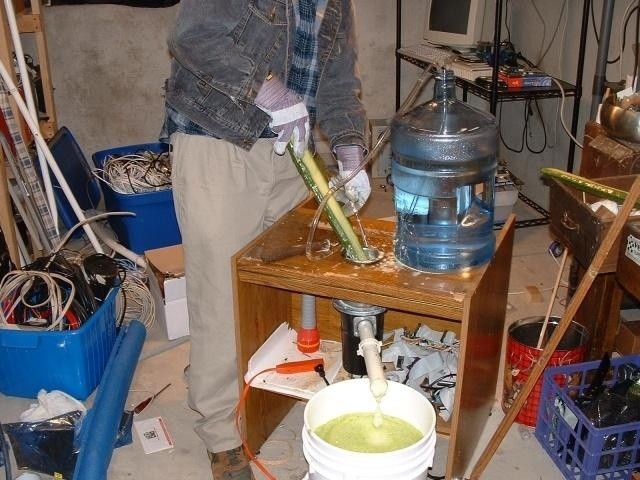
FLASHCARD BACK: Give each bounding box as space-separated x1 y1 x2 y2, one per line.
252 73 311 160
328 143 372 219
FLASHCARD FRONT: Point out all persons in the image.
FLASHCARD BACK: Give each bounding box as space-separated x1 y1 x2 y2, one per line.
157 0 374 480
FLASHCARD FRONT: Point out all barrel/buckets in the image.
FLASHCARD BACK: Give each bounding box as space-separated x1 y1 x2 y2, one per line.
390 69 499 273
500 316 591 426
302 379 438 480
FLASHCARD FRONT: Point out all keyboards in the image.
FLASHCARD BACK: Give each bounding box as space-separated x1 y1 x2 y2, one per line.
397 44 456 68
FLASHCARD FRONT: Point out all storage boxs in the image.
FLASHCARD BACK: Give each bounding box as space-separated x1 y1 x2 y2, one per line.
143 242 190 342
0 278 121 403
32 127 182 254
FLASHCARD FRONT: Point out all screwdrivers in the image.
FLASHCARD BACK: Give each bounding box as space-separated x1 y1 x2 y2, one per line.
135 383 171 414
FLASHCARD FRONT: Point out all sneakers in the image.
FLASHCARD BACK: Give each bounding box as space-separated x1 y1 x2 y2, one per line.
184 363 254 479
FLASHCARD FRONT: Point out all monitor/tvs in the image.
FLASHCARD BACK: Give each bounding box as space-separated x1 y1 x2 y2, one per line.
423 0 512 58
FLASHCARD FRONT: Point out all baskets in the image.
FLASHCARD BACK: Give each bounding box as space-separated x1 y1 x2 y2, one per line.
534 355 640 480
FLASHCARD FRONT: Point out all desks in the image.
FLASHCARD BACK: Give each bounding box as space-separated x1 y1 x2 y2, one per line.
545 123 640 395
229 192 517 480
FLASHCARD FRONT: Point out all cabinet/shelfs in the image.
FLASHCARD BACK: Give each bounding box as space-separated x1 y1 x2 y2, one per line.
395 0 590 232
